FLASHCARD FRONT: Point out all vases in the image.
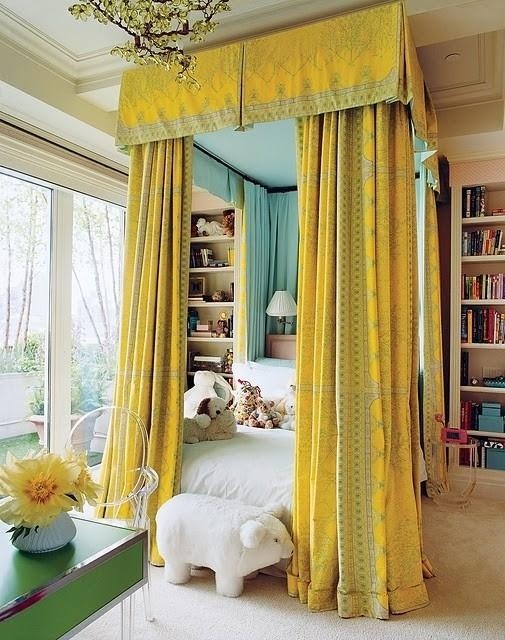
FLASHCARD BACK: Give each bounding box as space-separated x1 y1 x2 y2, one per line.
13 512 76 553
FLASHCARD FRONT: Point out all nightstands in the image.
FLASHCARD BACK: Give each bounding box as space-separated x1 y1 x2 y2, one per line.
266 333 296 359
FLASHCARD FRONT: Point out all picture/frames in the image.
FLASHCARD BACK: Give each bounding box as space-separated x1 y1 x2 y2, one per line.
190 277 206 296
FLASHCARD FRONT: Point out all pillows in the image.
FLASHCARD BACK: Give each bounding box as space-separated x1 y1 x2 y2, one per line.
231 358 296 401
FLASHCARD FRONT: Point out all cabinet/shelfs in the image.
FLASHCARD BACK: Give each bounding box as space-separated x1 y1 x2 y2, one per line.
448 149 505 472
185 207 237 391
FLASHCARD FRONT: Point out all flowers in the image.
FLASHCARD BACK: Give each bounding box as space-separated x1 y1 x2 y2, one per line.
0 449 103 544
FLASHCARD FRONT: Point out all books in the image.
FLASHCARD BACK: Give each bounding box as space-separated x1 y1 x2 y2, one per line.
461 352 469 385
190 247 213 266
461 273 505 344
460 400 481 431
462 186 505 256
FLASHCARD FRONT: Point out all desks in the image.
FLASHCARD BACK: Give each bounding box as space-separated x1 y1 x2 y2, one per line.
426 435 479 508
0 512 148 640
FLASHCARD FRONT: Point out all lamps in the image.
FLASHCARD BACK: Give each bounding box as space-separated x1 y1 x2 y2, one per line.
265 290 297 334
68 0 231 96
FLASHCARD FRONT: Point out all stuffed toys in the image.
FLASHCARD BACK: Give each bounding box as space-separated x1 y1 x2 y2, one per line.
155 493 295 598
196 213 234 237
184 370 296 443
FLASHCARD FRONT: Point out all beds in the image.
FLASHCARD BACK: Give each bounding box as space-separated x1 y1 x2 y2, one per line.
183 425 428 577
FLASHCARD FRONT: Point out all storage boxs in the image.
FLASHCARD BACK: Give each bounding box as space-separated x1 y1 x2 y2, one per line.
487 448 505 470
482 403 501 416
479 415 504 432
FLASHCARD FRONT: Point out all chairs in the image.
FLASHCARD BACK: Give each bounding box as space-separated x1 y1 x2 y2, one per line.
62 406 159 640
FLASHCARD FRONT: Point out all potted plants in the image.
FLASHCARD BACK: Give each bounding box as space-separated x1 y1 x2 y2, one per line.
15 333 113 454
